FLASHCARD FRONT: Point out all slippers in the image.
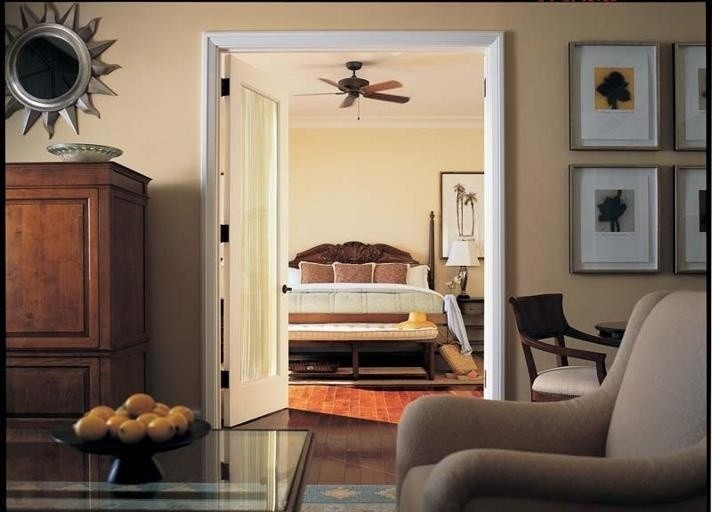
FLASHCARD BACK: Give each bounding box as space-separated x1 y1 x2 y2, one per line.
445 371 465 378
457 370 483 381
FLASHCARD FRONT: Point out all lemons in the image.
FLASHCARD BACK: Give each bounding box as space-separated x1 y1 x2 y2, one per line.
71 392 195 447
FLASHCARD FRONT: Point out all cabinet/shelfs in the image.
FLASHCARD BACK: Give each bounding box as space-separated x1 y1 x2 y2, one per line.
5 161 153 417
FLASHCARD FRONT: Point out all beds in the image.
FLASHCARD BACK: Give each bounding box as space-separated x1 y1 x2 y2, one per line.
289 240 447 357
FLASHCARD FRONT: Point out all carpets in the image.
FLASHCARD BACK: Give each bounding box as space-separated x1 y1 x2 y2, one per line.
6 481 396 512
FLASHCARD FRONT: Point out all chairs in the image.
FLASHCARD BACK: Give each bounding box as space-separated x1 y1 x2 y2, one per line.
508 293 620 402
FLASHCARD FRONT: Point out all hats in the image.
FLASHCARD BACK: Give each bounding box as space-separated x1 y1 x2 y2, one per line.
398 311 436 329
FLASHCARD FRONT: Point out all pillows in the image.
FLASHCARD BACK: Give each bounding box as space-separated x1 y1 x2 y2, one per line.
298 260 334 283
331 261 376 283
374 263 410 284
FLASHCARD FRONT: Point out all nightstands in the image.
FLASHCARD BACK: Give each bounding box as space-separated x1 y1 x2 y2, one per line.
445 299 484 353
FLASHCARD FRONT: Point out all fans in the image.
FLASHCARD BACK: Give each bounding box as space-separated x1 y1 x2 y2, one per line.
291 61 411 120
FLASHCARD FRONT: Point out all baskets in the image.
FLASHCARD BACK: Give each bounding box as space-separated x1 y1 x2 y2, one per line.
436 341 478 372
289 360 339 372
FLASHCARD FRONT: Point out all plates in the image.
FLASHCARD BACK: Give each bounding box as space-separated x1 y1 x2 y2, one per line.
46 143 123 163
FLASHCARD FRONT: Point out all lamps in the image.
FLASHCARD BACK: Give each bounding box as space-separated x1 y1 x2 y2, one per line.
445 241 481 299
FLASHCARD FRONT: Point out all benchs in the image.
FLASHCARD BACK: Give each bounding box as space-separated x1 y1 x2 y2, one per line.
287 324 437 381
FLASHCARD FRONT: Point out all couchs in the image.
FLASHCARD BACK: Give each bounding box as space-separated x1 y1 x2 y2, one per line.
394 289 707 512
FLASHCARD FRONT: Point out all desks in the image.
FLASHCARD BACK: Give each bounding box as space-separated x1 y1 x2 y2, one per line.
594 321 626 341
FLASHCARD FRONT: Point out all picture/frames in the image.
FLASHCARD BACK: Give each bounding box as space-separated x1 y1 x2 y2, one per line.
569 163 661 275
671 41 706 152
439 171 484 260
568 40 663 152
672 164 707 275
